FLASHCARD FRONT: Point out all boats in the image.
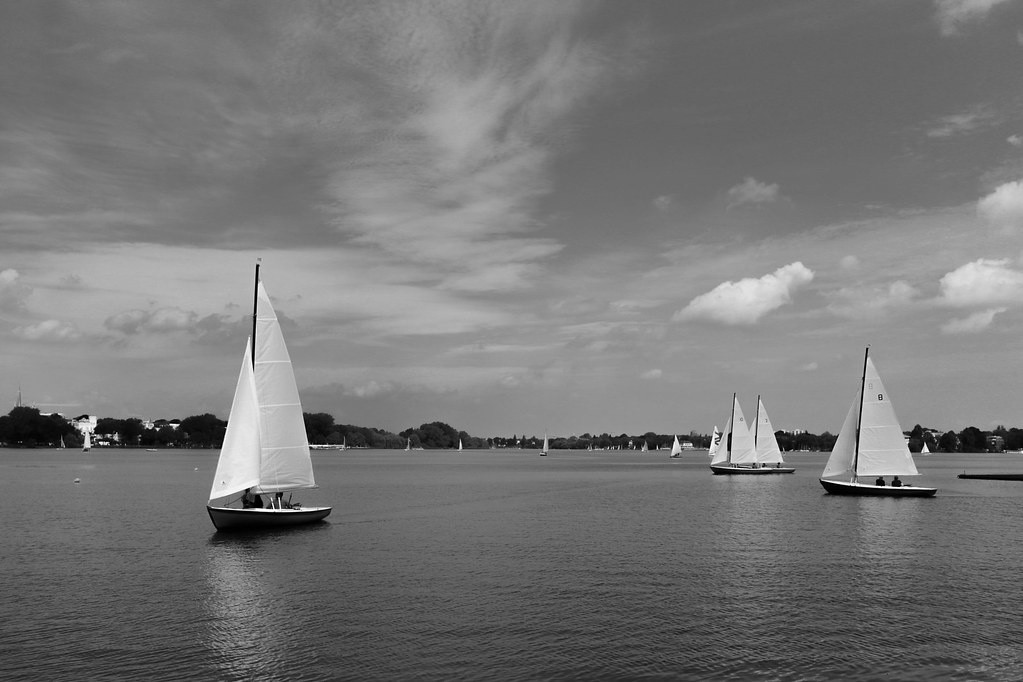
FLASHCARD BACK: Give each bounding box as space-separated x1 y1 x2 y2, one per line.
308 444 345 450
146 448 158 453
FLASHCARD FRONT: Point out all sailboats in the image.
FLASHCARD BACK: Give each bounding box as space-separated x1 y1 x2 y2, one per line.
81 427 91 454
56 434 66 450
920 441 930 455
457 438 464 452
540 434 549 456
708 425 720 457
820 346 937 498
204 256 334 534
749 394 796 473
709 394 772 475
585 440 650 454
403 437 425 452
669 434 683 458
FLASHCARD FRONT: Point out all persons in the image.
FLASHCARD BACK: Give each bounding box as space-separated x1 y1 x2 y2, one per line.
267 492 291 509
762 463 767 467
752 463 757 469
891 476 902 487
876 476 885 486
777 462 781 468
242 488 263 508
